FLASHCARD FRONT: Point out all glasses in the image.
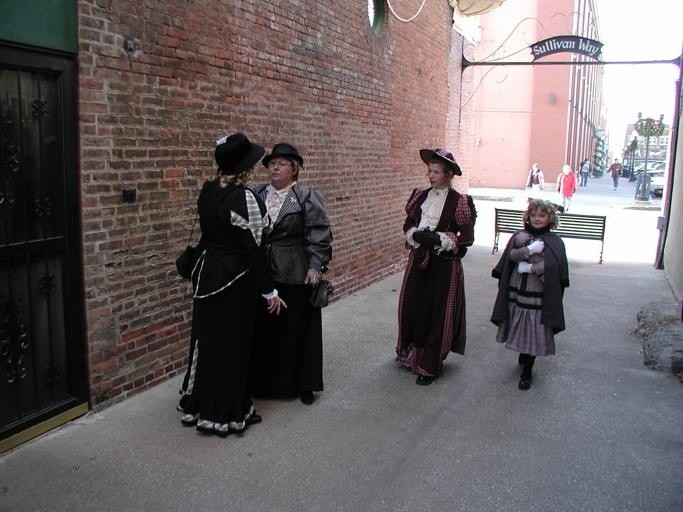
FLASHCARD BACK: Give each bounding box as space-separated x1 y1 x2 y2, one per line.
268 159 293 169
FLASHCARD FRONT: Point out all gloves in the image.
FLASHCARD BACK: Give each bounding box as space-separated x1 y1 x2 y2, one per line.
527 240 545 255
518 261 531 274
412 228 441 246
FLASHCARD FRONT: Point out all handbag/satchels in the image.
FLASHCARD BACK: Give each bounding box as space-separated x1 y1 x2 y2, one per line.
176 210 199 281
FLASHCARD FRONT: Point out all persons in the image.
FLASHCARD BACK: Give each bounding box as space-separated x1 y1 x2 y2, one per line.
489 196 570 392
176 133 287 439
578 158 592 187
605 158 623 192
554 164 575 212
249 142 334 404
524 163 545 191
393 147 478 387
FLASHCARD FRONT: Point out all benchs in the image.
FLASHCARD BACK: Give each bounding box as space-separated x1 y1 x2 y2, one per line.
490 206 607 267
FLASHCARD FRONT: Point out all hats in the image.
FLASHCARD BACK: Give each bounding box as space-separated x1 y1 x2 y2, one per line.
215 133 265 176
419 148 462 176
261 143 303 169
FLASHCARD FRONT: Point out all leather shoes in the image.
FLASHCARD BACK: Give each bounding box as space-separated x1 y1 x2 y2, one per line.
416 373 435 385
518 370 531 389
300 391 313 405
246 415 262 425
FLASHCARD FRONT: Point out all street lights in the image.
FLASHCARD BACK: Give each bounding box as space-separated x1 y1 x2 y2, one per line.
621 136 638 182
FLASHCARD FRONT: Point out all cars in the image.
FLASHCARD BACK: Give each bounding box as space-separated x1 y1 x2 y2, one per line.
624 159 665 198
